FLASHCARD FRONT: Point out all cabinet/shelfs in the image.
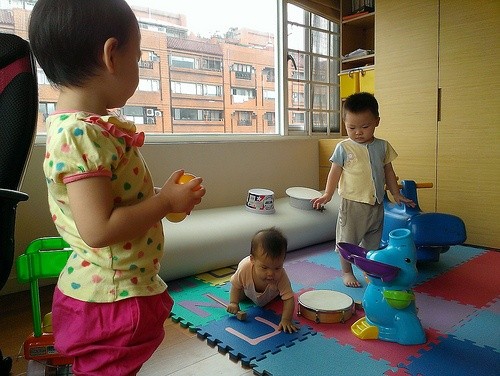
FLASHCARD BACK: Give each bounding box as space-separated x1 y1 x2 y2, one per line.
338 0 378 140
374 0 500 253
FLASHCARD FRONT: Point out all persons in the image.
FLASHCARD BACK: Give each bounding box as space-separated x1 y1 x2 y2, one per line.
227 226 301 334
28 0 204 376
310 91 416 287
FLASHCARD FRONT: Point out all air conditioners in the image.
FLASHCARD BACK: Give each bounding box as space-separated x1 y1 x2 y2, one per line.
146 117 154 124
155 111 161 116
145 109 155 117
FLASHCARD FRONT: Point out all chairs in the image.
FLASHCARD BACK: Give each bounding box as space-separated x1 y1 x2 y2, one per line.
0 31 40 376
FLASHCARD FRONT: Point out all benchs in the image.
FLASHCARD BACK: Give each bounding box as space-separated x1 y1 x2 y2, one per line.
138 134 340 304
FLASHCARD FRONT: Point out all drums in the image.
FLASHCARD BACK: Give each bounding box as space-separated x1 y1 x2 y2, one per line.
298 289 354 325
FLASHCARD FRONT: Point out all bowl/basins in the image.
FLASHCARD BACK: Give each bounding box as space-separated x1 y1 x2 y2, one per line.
245 188 276 214
285 186 322 209
